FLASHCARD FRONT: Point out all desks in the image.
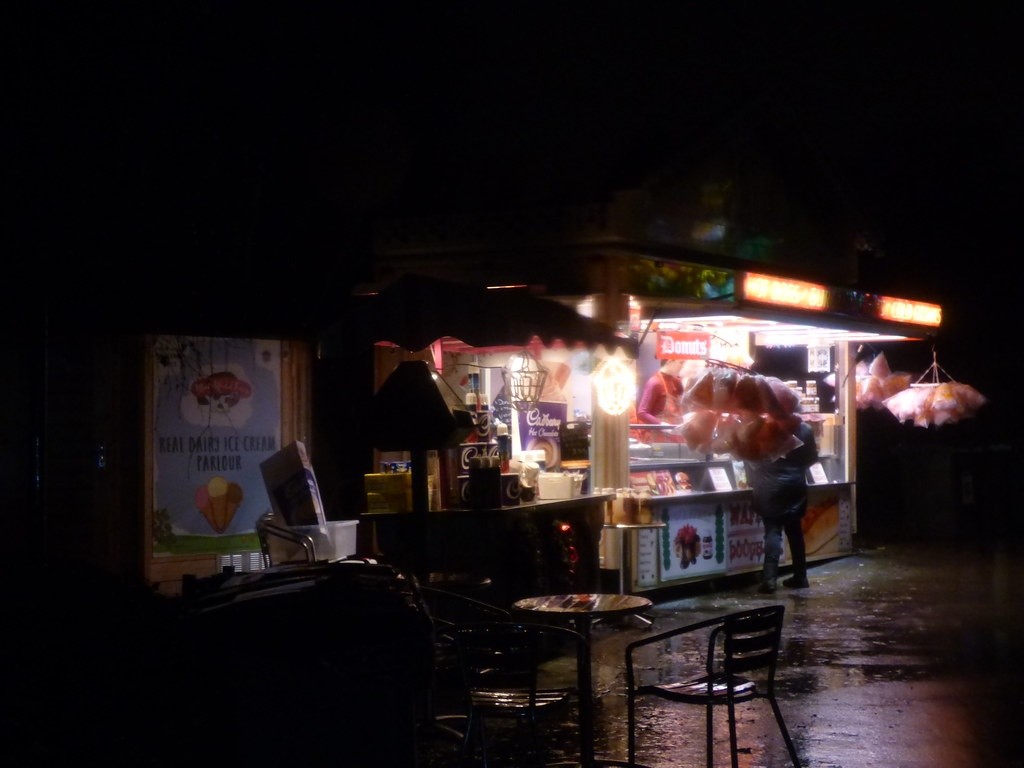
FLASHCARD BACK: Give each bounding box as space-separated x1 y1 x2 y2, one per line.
603 521 665 627
512 593 652 768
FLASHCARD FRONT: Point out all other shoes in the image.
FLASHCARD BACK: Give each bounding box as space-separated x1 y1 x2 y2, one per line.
757 578 777 594
782 576 809 588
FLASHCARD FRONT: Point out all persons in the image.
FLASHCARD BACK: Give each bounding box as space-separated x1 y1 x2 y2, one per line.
743 386 818 592
638 352 685 444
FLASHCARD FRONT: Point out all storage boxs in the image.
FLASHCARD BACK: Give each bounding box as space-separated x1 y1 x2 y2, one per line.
259 441 326 526
264 519 359 563
365 473 413 512
536 472 583 498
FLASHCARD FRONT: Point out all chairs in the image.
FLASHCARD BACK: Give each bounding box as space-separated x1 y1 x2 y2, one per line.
444 605 802 768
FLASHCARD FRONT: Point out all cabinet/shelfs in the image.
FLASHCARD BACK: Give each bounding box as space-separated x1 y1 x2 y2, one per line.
630 422 857 504
